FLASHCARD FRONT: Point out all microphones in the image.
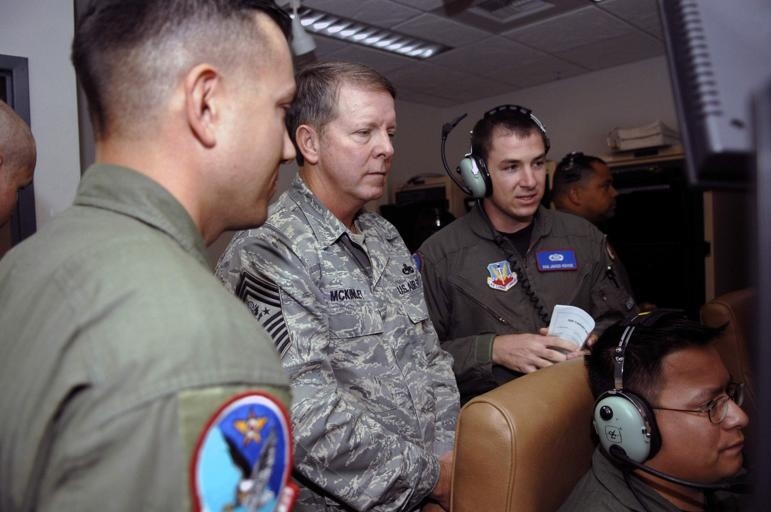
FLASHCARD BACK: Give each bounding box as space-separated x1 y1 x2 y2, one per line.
626 453 746 500
436 110 472 195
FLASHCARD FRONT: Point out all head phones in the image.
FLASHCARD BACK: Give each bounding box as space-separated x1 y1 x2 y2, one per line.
590 304 663 472
460 100 552 204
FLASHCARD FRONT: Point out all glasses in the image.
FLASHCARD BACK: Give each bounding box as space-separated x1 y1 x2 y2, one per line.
651 381 745 424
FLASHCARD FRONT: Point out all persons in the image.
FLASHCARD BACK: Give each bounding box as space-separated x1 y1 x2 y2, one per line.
414 107 644 411
554 309 771 512
211 58 464 511
0 0 300 512
542 152 617 228
0 98 36 231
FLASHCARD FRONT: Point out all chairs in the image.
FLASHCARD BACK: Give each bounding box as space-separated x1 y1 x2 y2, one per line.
450 340 617 511
698 285 765 473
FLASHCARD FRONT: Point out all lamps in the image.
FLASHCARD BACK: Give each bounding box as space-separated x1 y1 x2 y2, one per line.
286 1 318 58
